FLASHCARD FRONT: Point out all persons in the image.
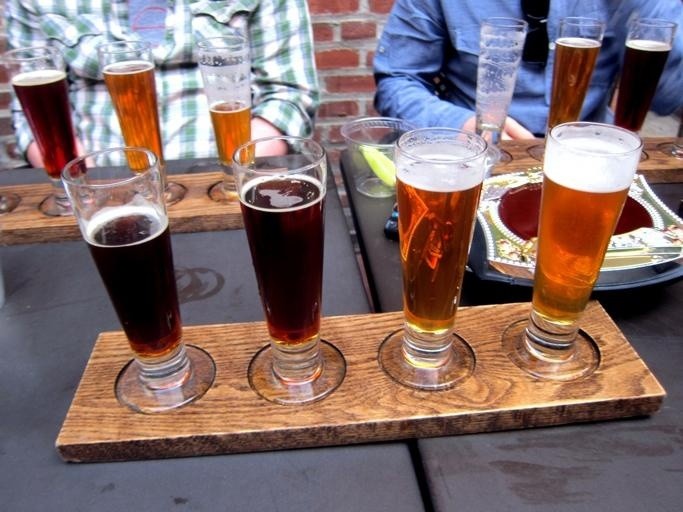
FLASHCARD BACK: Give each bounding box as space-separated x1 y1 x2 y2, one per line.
373 0 682 143
0 0 321 169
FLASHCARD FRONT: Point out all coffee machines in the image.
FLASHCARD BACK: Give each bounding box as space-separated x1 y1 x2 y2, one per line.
500 117 646 385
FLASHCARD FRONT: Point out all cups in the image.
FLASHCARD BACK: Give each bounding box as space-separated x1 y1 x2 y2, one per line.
0 45 93 216
475 14 676 165
94 41 188 210
232 134 348 410
57 147 216 415
196 33 251 205
378 125 488 395
339 115 415 199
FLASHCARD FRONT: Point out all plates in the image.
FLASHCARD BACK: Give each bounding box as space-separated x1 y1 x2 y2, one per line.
466 166 682 292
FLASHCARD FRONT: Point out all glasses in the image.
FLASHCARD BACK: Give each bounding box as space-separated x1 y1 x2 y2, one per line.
521 1 550 72
